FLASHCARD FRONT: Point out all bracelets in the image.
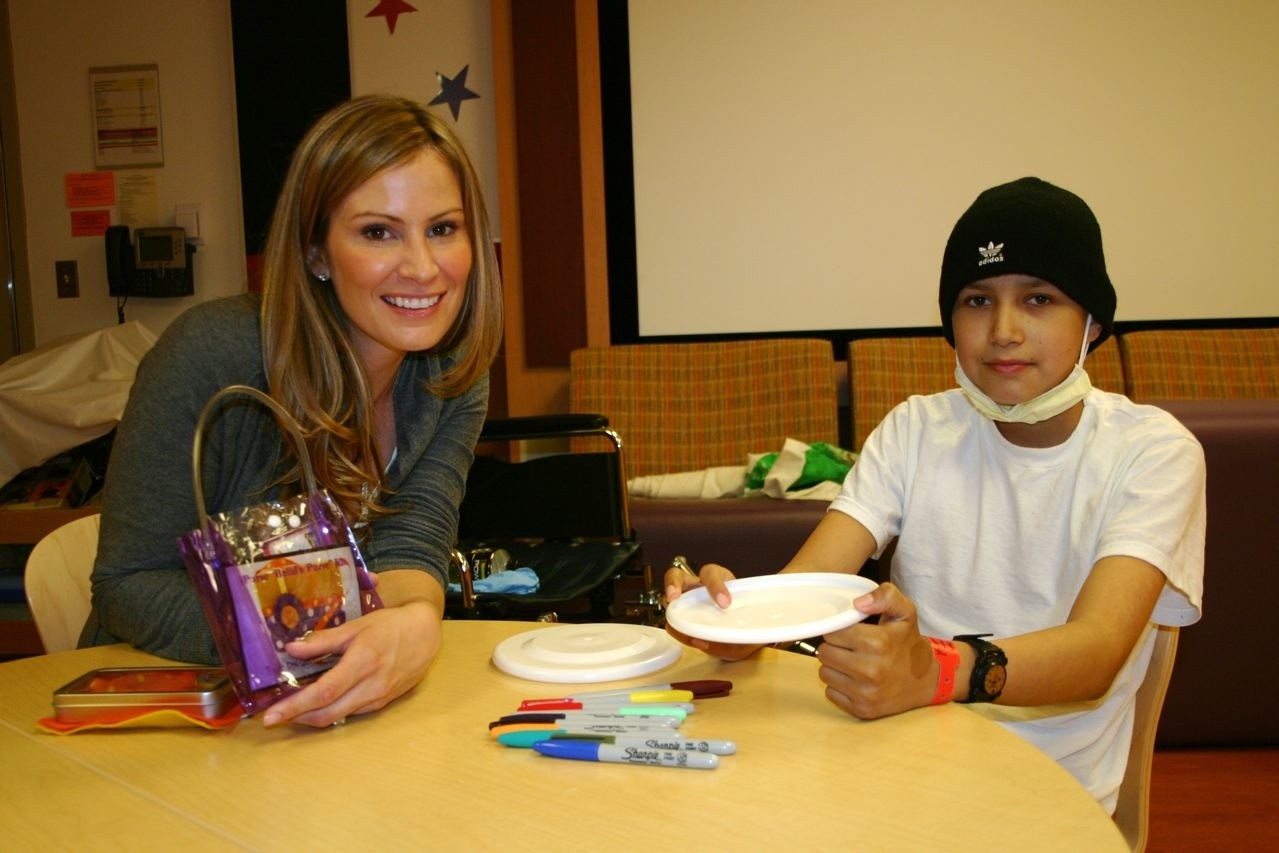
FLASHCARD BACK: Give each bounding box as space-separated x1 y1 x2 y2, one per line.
927 637 960 707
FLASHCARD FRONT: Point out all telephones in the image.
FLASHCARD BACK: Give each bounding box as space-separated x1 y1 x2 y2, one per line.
104 224 197 324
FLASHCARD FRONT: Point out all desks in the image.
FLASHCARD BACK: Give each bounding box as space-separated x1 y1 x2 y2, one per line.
0 618 1130 853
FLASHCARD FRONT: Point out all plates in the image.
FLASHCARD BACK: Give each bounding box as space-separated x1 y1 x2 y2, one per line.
492 623 681 683
665 571 885 645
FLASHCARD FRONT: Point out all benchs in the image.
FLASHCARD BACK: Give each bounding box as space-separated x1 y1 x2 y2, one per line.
571 308 1279 478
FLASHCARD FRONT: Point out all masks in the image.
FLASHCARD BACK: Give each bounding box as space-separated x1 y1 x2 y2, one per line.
955 312 1093 424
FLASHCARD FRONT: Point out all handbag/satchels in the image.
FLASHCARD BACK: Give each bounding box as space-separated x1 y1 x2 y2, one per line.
177 385 384 717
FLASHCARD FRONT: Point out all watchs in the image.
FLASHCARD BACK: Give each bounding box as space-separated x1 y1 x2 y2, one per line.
952 633 1008 703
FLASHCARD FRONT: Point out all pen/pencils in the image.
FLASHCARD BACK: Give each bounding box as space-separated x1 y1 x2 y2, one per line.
488 678 737 771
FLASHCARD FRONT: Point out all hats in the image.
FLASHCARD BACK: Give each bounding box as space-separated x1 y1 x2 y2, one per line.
939 176 1117 354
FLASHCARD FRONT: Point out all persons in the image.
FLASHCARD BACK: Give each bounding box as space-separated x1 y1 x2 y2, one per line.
78 93 504 731
664 176 1206 818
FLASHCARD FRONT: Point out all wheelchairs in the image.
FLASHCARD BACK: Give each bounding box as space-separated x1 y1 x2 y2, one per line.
444 411 665 630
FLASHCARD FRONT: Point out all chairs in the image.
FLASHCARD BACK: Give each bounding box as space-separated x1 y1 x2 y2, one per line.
24 513 103 650
447 407 640 619
1117 623 1184 853
